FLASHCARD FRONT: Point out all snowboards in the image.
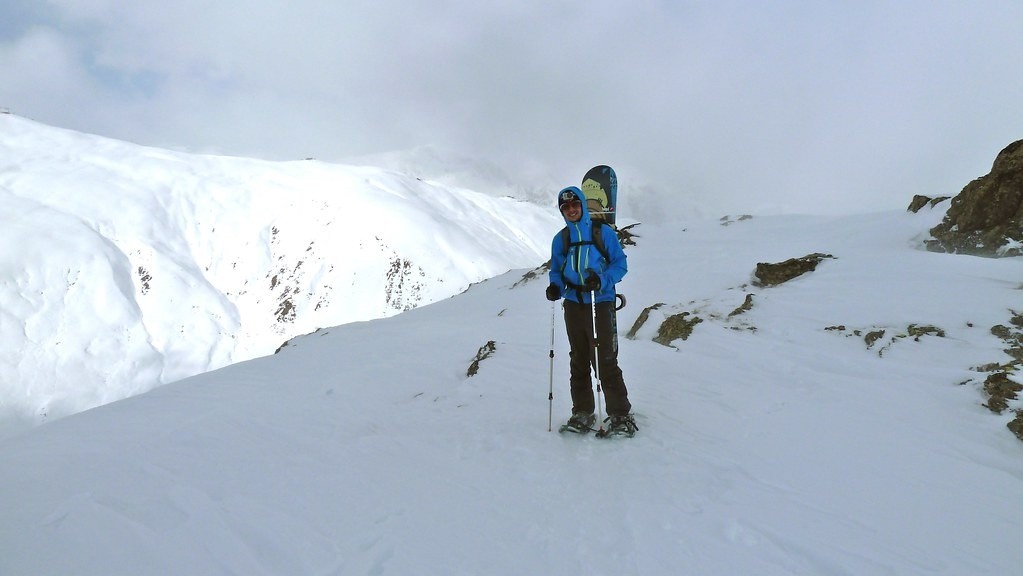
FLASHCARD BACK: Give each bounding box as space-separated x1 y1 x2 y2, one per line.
581 166 618 231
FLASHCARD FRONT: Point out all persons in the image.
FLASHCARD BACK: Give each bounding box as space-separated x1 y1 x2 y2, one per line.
546 186 636 433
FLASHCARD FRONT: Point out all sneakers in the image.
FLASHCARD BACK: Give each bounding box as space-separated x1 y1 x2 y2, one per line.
567 411 596 433
608 413 636 439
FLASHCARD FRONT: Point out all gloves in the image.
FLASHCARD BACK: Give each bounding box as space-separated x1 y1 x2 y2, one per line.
584 271 600 292
545 285 561 301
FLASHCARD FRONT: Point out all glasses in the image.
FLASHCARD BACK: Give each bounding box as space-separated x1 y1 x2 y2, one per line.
561 202 581 211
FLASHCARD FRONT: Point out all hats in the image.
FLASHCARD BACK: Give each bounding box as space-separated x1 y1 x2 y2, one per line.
559 190 580 210
